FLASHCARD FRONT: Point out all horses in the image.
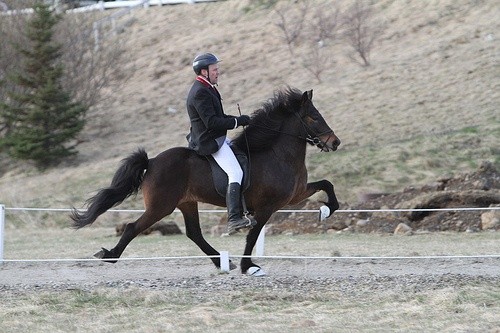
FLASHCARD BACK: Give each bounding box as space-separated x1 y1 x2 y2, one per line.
67 83 341 277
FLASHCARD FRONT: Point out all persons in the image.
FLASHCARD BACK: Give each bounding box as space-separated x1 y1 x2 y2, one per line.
186 52 257 235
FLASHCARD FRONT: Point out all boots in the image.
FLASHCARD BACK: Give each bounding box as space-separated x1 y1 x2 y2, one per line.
226 182 257 235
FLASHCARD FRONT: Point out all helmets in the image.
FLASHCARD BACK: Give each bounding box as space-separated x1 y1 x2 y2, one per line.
193 53 223 75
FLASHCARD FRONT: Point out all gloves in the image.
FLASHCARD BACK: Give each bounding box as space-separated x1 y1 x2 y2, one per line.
236 115 250 128
